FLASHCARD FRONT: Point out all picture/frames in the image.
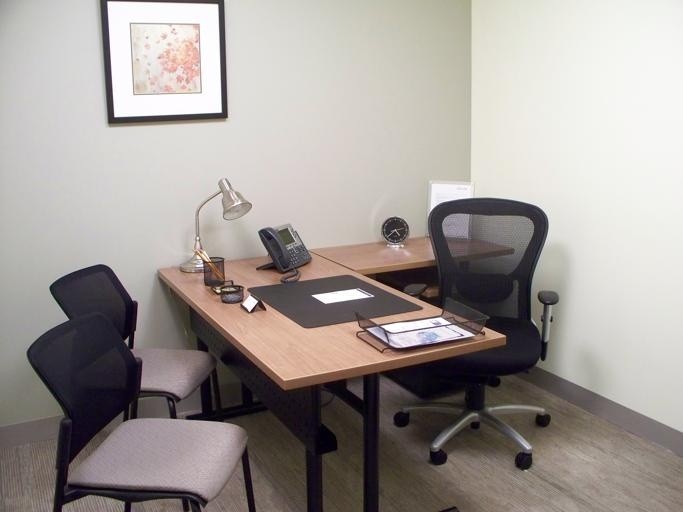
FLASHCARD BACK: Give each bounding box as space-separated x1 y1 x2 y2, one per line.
426 179 475 240
100 0 227 124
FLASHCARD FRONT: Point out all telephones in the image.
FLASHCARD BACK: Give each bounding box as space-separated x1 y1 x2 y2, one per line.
256 223 311 274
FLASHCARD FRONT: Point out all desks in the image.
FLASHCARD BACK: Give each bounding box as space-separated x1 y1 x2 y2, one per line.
159 248 508 512
312 236 513 425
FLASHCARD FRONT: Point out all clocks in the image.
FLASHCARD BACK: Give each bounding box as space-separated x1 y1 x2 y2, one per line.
381 216 409 248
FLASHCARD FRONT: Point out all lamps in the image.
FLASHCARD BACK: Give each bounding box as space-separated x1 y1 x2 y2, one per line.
179 177 252 273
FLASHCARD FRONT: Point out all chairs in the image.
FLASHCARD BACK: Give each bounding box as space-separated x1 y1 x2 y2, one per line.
49 263 223 420
393 198 558 472
26 309 254 512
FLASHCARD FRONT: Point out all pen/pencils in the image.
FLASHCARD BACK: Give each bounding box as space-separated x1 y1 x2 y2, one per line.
192 248 224 280
